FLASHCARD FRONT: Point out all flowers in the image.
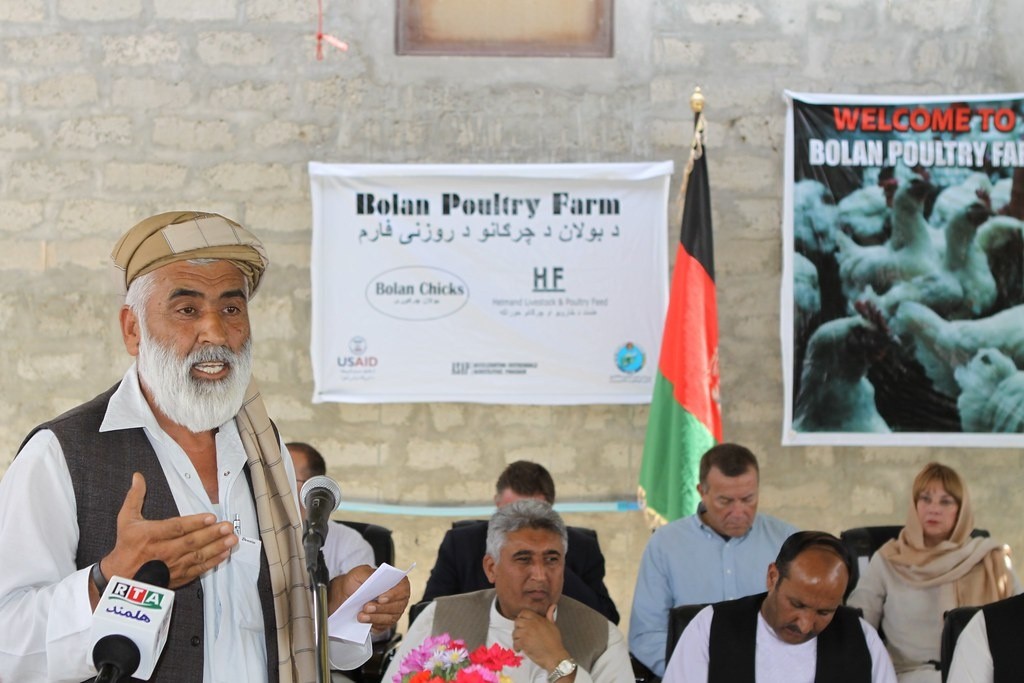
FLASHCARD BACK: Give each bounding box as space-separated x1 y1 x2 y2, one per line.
391 633 525 683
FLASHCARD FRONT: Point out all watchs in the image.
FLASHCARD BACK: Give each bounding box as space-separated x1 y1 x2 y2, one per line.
546 655 577 683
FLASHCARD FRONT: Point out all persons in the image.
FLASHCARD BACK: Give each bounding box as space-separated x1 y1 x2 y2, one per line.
285 442 394 643
946 592 1024 683
627 443 801 677
845 462 1024 683
0 212 412 683
379 498 636 683
660 531 899 683
422 460 621 624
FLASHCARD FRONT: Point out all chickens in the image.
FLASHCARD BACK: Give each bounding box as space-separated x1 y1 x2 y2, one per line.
790 109 1023 435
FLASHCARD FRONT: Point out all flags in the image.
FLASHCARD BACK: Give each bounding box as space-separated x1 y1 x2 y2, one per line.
637 111 723 532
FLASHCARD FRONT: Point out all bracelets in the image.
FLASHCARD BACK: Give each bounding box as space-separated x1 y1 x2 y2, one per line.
91 560 108 599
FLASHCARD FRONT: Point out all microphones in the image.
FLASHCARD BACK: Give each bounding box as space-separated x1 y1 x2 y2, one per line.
299 475 343 574
86 559 177 683
